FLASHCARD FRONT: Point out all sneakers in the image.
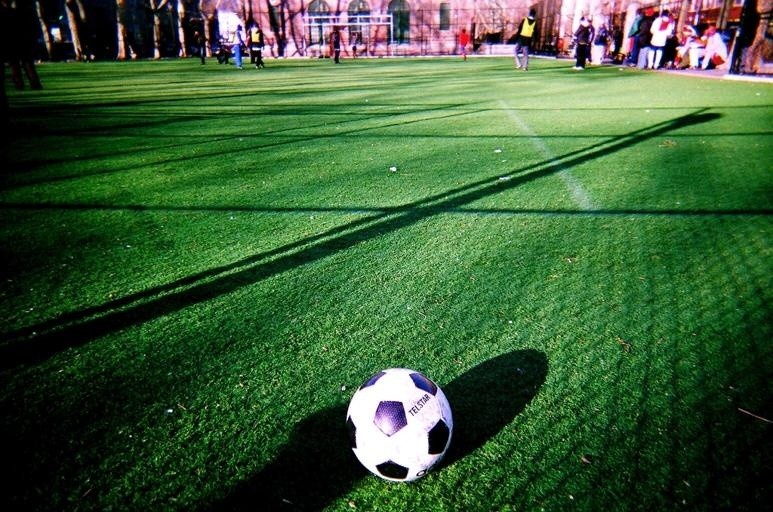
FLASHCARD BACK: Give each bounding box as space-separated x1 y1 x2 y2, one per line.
572 64 586 70
622 61 707 72
515 65 529 70
234 63 267 70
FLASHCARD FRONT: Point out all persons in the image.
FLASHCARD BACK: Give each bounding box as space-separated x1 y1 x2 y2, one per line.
334 31 340 63
514 8 538 70
192 21 265 69
353 42 358 59
0 0 42 89
459 29 468 61
574 0 760 74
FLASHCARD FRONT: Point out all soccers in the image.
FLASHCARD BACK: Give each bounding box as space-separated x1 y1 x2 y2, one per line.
345 368 454 482
